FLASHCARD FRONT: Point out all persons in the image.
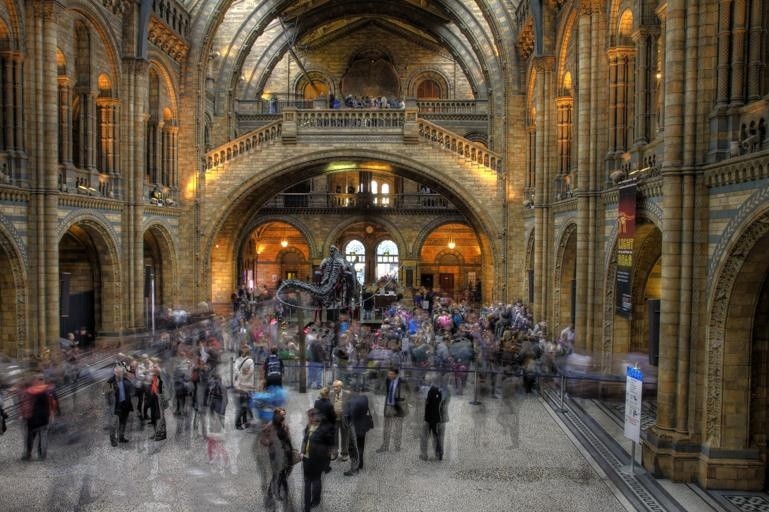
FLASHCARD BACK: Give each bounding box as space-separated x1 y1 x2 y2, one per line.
326 86 405 129
0 280 576 512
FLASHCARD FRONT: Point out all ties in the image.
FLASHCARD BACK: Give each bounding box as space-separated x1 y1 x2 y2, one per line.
388 381 395 404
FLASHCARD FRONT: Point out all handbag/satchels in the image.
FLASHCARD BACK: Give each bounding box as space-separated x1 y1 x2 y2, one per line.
393 398 409 418
354 412 373 432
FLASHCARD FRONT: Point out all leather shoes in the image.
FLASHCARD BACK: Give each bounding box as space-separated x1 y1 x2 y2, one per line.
375 444 402 455
110 437 130 447
147 434 166 441
343 469 359 477
